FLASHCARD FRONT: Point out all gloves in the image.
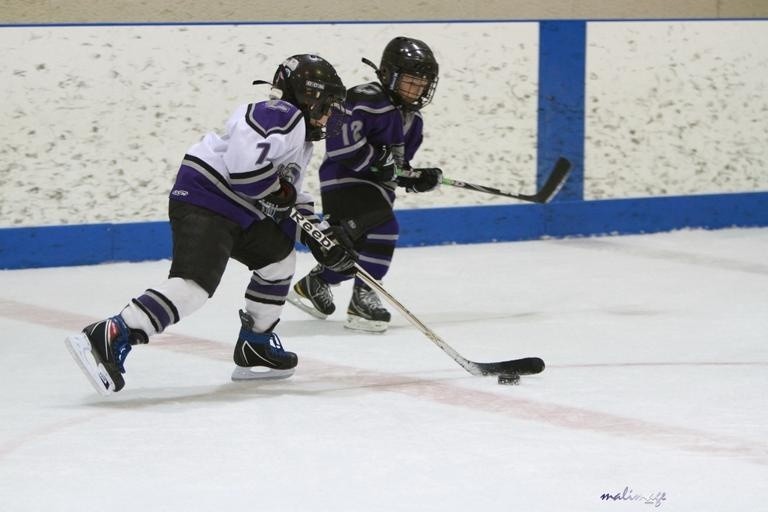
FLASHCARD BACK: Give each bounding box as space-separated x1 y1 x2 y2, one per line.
261 179 297 220
305 230 360 273
369 143 395 182
406 166 442 195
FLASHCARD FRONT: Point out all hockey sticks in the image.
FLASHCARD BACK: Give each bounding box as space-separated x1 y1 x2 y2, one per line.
397 157 571 203
290 208 545 377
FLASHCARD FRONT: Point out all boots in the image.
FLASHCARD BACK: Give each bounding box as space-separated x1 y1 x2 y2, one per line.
82 304 149 393
232 309 297 370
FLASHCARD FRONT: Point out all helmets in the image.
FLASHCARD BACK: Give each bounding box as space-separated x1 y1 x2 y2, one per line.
379 37 439 110
268 54 348 142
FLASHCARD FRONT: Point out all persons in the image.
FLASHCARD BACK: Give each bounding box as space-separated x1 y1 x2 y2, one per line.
293 36 444 321
84 54 361 393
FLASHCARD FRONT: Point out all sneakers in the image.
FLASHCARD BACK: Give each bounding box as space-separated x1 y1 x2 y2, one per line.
346 286 390 323
294 270 335 314
